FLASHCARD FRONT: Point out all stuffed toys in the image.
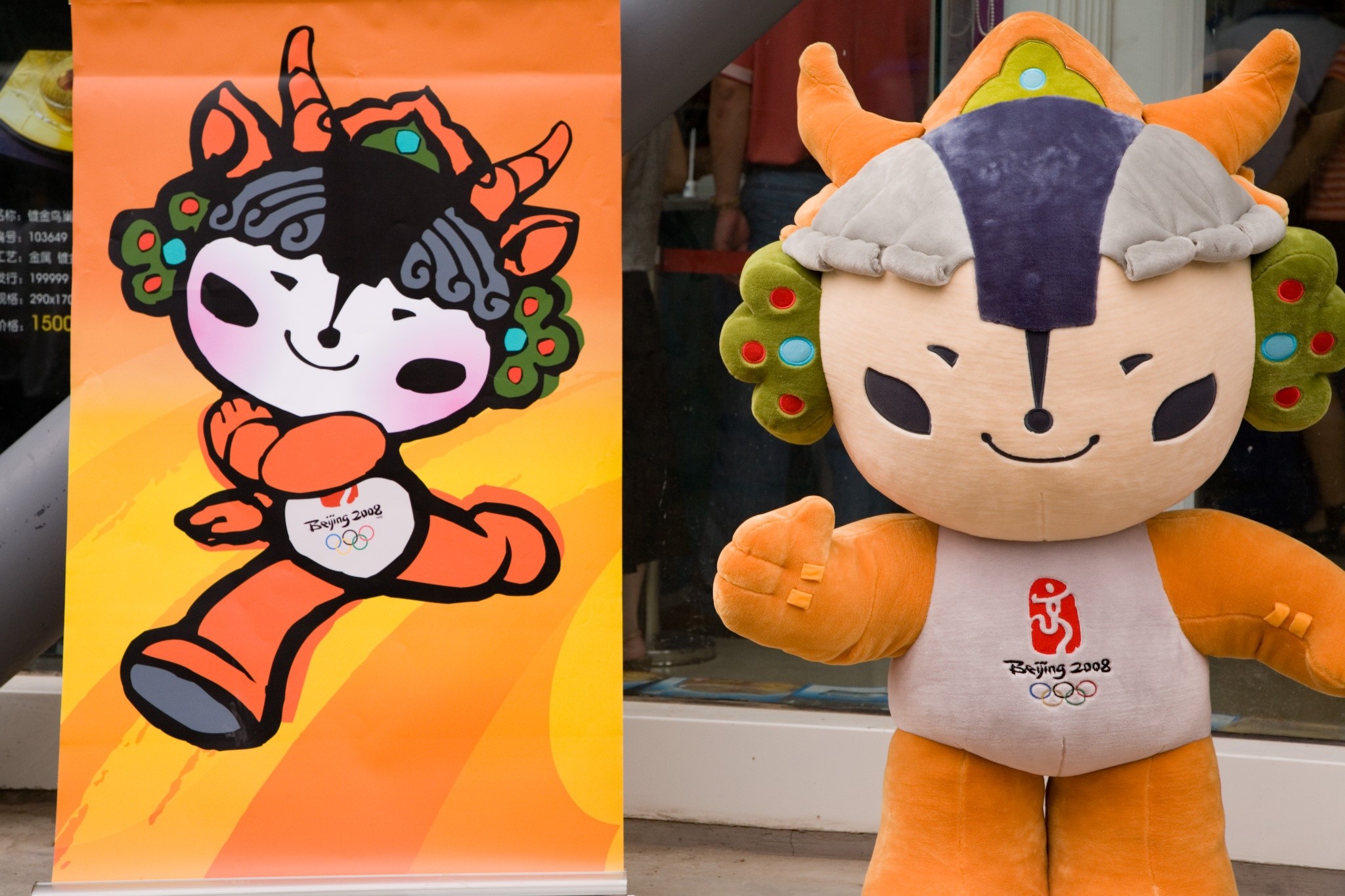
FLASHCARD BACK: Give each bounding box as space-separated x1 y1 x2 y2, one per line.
712 10 1345 896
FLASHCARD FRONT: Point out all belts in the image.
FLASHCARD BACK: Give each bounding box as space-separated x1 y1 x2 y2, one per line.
745 159 823 174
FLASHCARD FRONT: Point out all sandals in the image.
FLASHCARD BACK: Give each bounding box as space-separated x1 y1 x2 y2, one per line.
623 630 652 671
1278 501 1345 556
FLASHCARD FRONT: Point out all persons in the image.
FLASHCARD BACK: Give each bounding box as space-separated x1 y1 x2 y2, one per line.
622 0 932 672
1203 0 1345 567
